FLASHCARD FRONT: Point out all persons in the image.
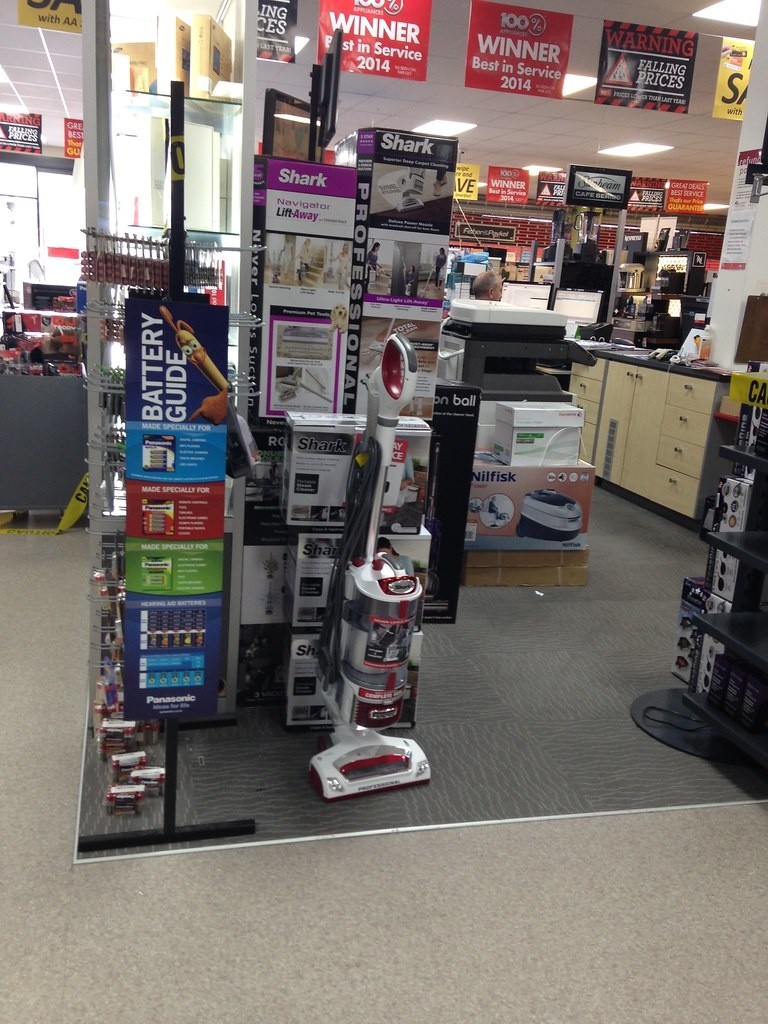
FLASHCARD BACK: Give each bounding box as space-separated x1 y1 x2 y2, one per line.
298 239 312 285
489 496 498 527
433 168 447 195
188 389 229 426
396 454 415 507
333 243 351 291
432 248 447 289
404 265 415 286
366 242 382 276
473 271 502 301
376 537 415 576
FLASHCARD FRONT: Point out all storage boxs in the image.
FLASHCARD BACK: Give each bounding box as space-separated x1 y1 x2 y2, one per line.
703 475 754 603
462 545 590 585
706 655 768 733
279 409 431 535
731 360 768 480
491 401 585 466
467 448 596 550
669 576 738 693
282 630 423 728
285 531 431 632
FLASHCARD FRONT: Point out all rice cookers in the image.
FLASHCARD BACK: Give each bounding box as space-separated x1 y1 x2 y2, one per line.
618 262 647 292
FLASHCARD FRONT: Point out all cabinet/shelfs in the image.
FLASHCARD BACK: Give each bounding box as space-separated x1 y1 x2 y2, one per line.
0 129 768 865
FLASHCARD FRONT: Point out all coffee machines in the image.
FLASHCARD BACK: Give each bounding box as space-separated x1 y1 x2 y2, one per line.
541 209 573 262
571 211 602 263
